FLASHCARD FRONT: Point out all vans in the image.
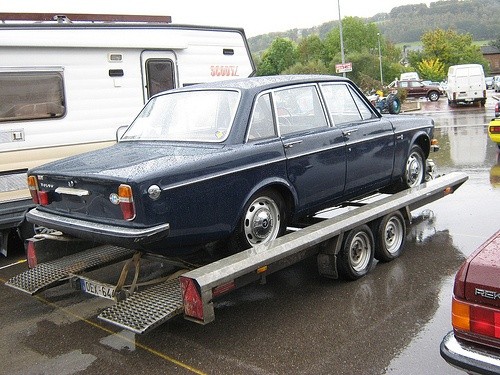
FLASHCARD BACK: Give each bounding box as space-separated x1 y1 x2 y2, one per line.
447 63 487 108
400 73 420 81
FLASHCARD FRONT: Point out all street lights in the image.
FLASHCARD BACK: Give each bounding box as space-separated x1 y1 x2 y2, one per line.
377 33 384 85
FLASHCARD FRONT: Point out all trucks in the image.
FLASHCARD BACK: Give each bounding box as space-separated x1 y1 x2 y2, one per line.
0 23 257 257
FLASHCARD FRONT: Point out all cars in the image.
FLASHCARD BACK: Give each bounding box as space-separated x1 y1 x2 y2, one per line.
25 74 435 248
486 76 500 93
440 230 500 374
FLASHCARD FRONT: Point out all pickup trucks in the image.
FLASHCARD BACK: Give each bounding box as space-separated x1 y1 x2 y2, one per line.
389 80 443 102
